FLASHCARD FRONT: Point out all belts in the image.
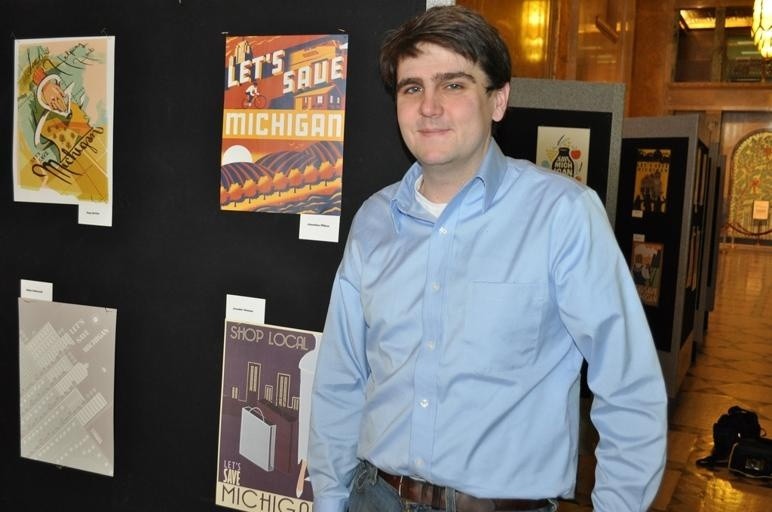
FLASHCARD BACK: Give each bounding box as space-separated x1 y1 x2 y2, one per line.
378 474 549 511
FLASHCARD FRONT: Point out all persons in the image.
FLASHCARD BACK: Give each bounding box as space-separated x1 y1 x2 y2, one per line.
306 6 669 511
633 194 665 212
631 253 650 285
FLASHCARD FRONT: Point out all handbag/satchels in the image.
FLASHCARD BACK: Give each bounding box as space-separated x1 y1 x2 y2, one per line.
239 406 276 472
728 438 772 479
695 406 766 468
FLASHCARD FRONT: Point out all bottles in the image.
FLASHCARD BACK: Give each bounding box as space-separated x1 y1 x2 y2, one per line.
552 147 575 178
